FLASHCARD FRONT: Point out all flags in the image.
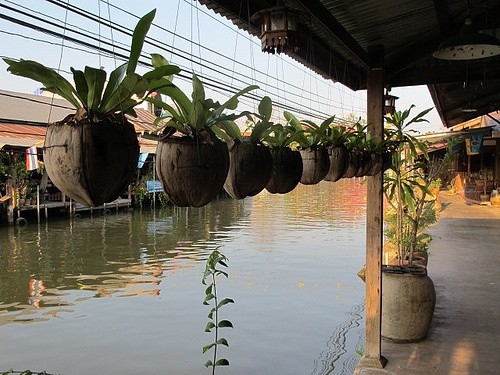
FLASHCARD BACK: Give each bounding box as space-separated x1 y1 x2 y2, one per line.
24 146 39 171
463 133 484 156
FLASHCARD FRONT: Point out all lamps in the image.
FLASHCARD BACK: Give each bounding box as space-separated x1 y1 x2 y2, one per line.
246 0 307 55
380 84 400 117
431 0 500 61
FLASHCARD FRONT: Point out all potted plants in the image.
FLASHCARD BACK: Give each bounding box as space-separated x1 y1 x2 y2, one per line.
364 101 444 343
2 7 404 208
